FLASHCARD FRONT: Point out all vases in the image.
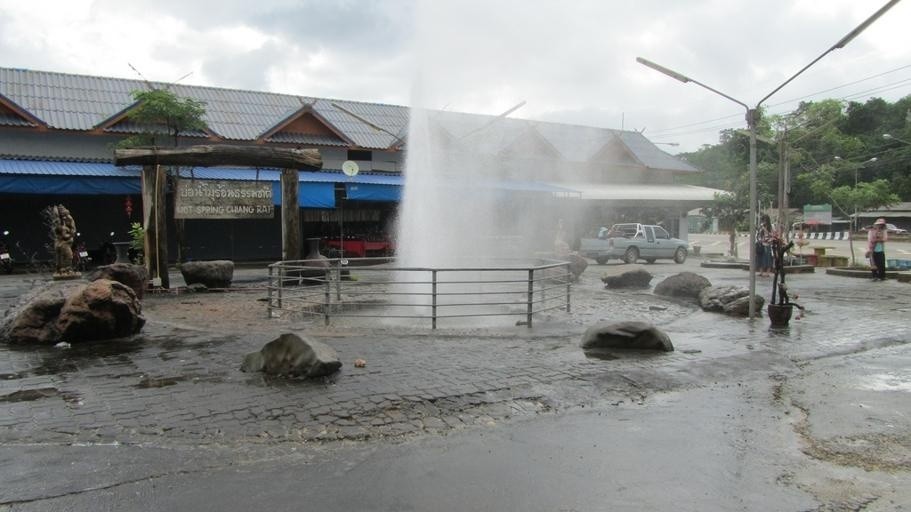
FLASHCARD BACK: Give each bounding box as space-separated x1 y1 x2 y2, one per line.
766 301 793 326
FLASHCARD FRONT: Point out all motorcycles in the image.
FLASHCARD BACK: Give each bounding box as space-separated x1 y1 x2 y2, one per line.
0 230 136 272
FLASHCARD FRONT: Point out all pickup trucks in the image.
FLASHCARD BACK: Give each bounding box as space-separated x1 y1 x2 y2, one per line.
578 221 702 262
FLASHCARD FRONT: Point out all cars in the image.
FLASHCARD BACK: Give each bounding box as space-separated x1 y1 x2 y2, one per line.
864 222 910 236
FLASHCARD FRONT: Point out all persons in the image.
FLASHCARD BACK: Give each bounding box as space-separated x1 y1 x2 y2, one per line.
50 206 75 275
755 214 776 277
868 217 890 282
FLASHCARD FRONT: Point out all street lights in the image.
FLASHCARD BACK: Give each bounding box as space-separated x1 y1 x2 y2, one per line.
882 133 911 146
835 155 878 232
735 116 839 239
329 98 529 157
634 0 902 320
